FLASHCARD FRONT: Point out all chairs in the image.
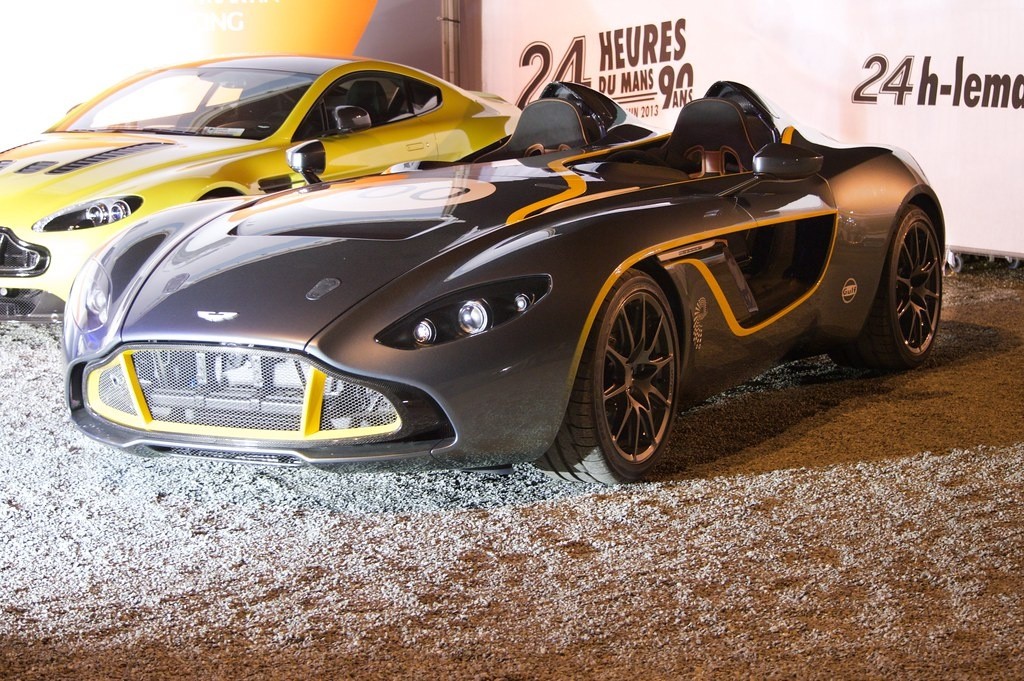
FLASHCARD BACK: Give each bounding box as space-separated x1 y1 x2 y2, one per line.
345 80 386 129
648 97 756 179
473 98 589 163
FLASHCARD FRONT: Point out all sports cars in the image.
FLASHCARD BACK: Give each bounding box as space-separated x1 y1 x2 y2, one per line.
62 74 949 489
0 51 524 324
